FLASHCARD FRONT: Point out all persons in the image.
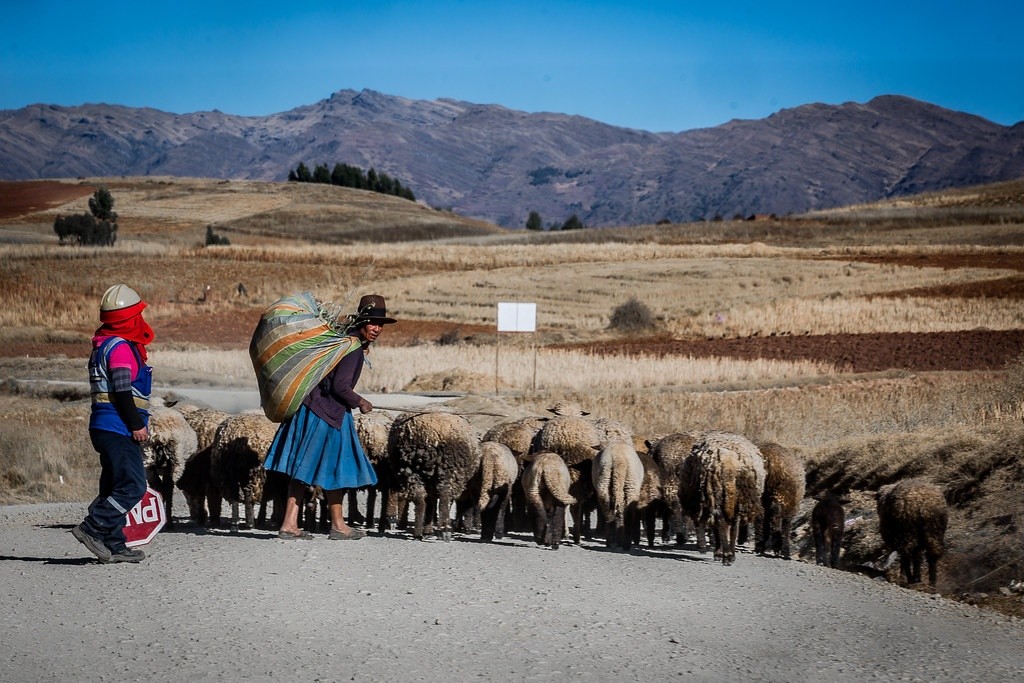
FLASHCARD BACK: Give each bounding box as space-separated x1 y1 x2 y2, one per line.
72 286 151 564
264 295 399 540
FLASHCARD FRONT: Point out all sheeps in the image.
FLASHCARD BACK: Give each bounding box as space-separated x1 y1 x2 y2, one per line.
872 479 948 591
810 491 852 569
139 396 199 529
178 400 803 567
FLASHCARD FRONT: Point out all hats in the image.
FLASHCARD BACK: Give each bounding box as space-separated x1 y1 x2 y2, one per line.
345 294 398 324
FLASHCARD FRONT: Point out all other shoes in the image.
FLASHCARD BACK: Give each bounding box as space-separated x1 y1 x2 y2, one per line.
330 527 365 539
278 530 313 540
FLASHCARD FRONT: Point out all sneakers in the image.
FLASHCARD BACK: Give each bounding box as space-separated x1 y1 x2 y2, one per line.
98 547 145 564
71 525 112 561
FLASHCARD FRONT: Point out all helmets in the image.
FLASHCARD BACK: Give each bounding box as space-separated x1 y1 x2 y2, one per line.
95 283 154 345
